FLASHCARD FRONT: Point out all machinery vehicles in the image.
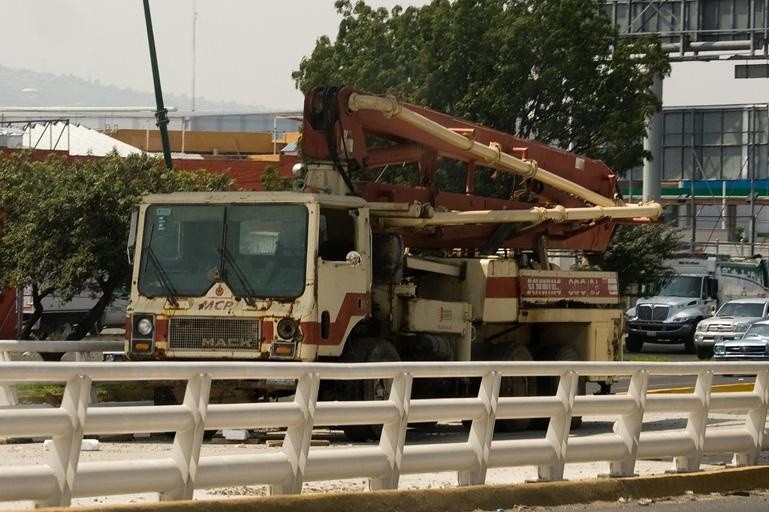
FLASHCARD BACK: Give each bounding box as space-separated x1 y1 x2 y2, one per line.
122 84 662 440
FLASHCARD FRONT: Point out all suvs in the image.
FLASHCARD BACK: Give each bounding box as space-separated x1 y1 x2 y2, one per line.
691 298 769 376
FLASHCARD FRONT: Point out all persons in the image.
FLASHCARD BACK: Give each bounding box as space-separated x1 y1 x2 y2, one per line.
319 239 363 266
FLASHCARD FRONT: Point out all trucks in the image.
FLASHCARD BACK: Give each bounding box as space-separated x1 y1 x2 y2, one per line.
626 258 764 352
